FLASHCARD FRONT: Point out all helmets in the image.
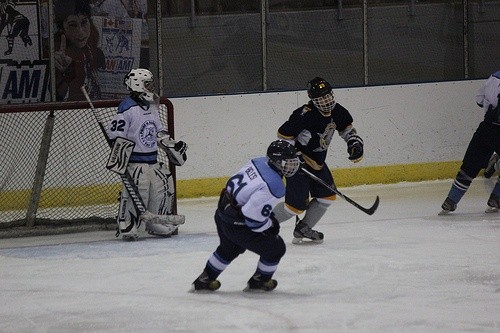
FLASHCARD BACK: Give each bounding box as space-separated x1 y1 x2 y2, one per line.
267 140 301 178
124 68 156 105
306 77 337 113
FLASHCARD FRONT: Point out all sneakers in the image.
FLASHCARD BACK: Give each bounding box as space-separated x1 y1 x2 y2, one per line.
242 266 277 294
485 192 500 214
437 197 457 216
292 220 324 245
188 264 221 293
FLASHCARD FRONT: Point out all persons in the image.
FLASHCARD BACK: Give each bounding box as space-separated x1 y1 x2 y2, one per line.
271 77 365 244
439 72 500 216
192 140 302 297
105 69 188 241
50 0 107 101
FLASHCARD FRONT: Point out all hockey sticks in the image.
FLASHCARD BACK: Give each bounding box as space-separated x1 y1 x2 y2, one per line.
298 164 380 216
80 86 186 224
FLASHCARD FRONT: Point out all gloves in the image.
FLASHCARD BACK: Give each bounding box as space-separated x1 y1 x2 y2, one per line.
292 146 309 177
158 129 188 167
346 135 364 162
